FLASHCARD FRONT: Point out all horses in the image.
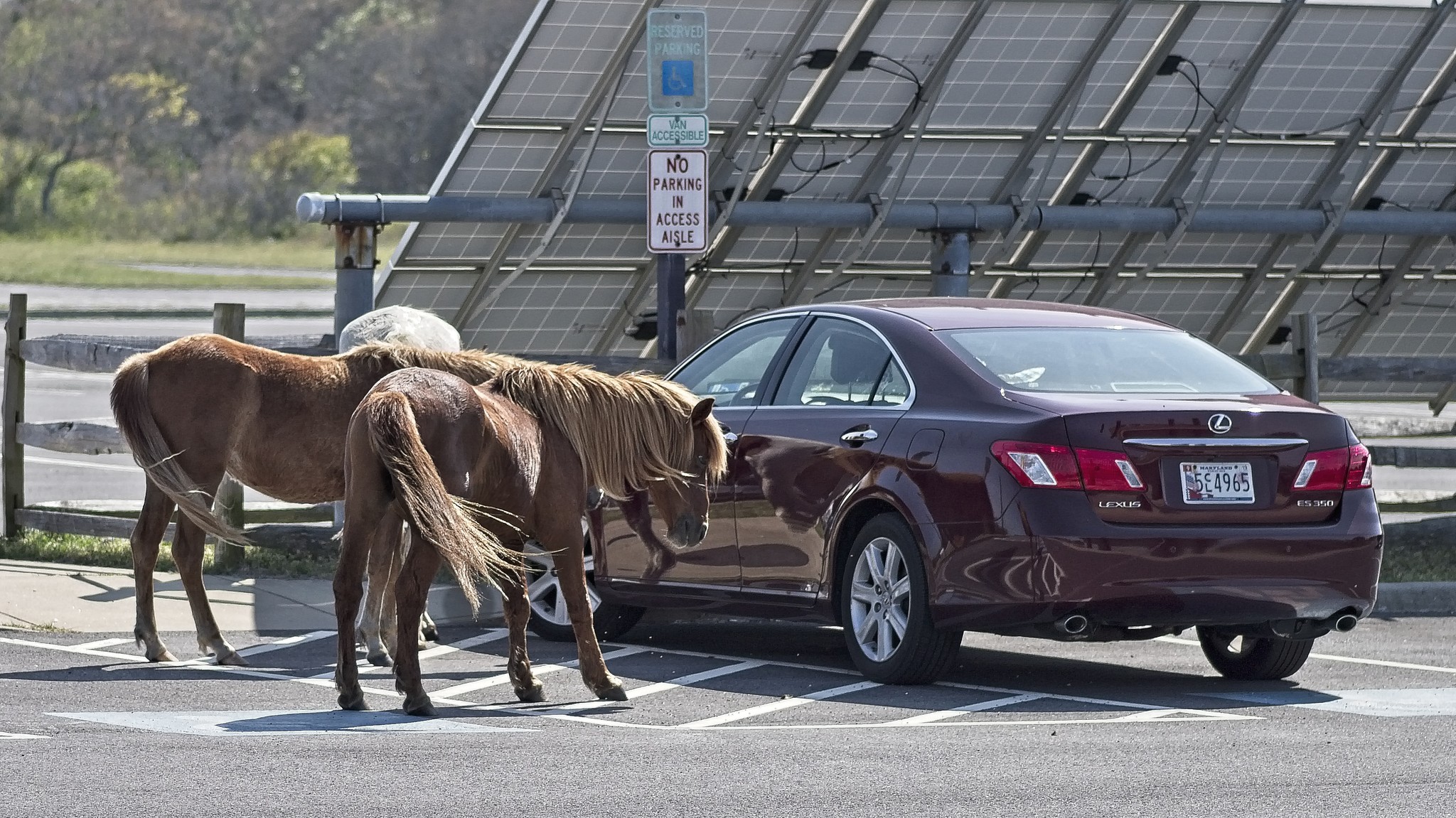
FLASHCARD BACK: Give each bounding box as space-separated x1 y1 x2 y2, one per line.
112 332 555 665
333 358 727 714
332 304 462 666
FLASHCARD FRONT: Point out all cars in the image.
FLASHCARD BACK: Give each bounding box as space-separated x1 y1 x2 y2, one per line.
514 292 1384 689
